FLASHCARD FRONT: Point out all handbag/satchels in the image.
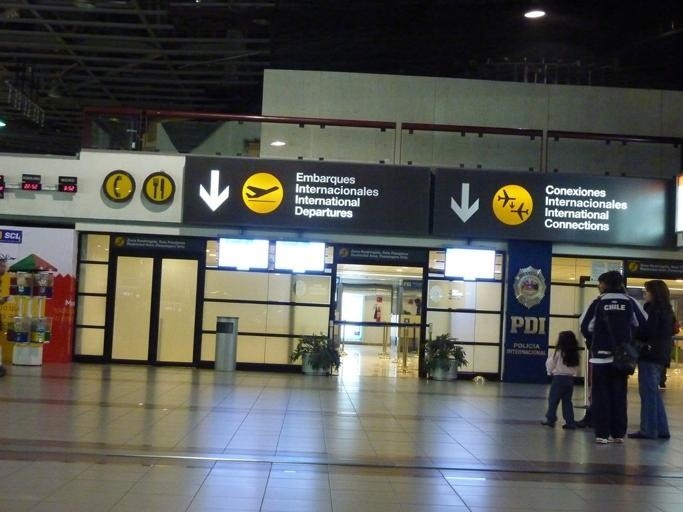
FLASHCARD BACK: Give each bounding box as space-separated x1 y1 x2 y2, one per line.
612 349 637 375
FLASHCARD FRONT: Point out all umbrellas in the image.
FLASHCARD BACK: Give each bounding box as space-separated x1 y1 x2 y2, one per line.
7 252 57 297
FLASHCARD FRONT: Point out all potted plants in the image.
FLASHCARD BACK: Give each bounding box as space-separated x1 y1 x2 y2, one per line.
290 331 348 377
415 332 470 382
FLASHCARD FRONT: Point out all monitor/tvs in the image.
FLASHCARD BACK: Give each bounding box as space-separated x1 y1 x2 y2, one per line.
218 238 270 271
445 248 496 282
275 239 326 273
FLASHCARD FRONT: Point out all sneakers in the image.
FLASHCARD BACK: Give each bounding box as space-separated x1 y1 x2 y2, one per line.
596 434 624 444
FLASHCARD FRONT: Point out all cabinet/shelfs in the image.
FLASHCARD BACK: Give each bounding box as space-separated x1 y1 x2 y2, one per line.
6 267 54 366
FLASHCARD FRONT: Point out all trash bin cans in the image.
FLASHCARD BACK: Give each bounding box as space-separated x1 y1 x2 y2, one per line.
215 316 239 372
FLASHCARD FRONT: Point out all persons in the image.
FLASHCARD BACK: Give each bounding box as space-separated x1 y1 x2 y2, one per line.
0 258 11 378
657 327 674 391
538 328 583 431
569 273 611 428
578 270 648 445
626 277 680 437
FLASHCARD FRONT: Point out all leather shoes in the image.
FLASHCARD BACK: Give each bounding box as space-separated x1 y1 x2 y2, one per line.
628 431 670 438
542 417 592 429
